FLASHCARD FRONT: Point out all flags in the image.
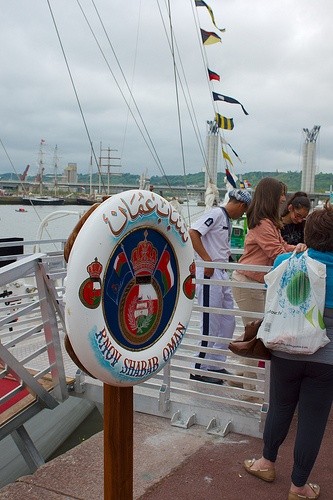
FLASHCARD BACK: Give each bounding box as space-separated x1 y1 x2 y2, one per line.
208 68 220 82
221 139 253 188
194 0 226 32
200 28 223 47
211 92 248 115
216 113 234 131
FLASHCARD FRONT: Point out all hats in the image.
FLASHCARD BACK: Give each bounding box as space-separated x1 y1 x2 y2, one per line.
229 188 252 204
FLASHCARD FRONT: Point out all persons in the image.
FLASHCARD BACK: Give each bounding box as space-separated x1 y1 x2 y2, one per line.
230 178 299 404
188 189 253 389
278 191 310 246
243 198 333 500
323 199 330 208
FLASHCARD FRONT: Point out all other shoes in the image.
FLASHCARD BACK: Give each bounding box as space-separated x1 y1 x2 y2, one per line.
288 482 320 500
189 368 234 384
243 458 275 482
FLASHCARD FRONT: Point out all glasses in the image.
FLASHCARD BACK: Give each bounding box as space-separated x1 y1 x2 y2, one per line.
292 206 307 221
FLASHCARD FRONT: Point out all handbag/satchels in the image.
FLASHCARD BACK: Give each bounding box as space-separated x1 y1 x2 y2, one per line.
257 246 330 356
228 319 272 360
